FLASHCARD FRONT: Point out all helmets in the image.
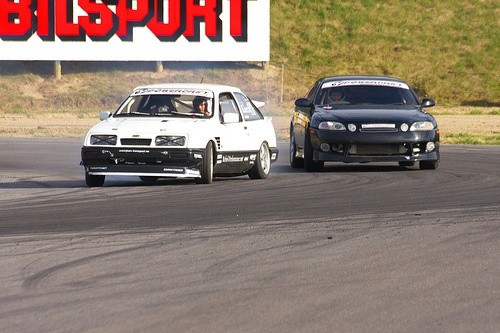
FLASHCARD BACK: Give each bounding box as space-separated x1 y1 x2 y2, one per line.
193 95 212 112
328 86 345 102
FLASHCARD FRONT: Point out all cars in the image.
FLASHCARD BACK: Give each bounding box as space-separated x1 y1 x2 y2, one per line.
289 75 441 172
79 82 279 188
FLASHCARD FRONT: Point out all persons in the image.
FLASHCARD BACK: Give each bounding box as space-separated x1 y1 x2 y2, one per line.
193 97 211 117
